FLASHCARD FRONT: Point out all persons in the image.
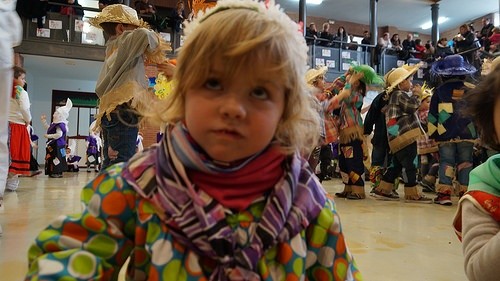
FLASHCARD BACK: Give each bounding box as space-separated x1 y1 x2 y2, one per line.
306 23 359 51
60 0 84 44
88 5 166 173
305 58 490 201
41 98 73 178
429 54 481 205
361 30 455 62
98 0 186 54
452 56 500 281
5 65 32 190
375 67 434 204
24 0 365 281
0 0 23 235
453 15 500 69
85 120 103 174
65 147 82 173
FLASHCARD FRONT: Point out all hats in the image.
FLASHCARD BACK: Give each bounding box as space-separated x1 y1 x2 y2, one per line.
88 4 149 31
481 56 500 74
347 63 383 86
431 53 477 75
385 61 421 94
419 80 435 102
305 65 328 84
488 33 500 42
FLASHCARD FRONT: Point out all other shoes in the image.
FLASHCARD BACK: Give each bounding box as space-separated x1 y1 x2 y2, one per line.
87 169 91 172
374 190 400 201
404 193 432 202
433 189 453 205
51 173 63 177
347 192 365 199
95 169 100 172
335 189 352 198
